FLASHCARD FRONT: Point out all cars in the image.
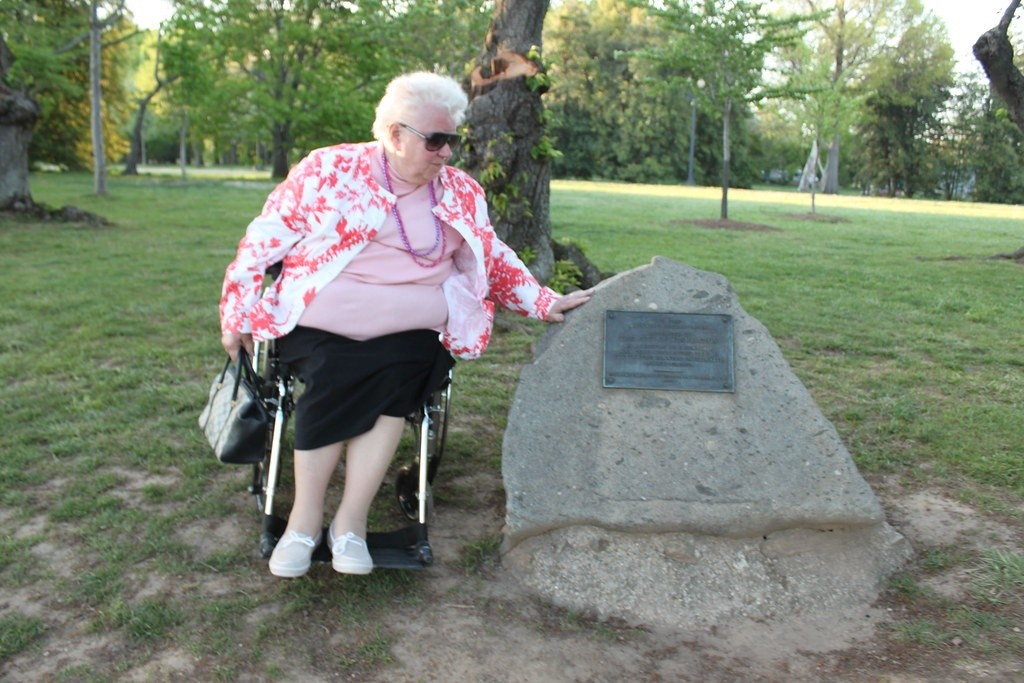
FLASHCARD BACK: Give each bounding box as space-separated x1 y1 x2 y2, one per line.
761 169 819 185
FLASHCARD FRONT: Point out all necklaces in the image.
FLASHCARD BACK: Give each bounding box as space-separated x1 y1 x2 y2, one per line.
383 148 448 268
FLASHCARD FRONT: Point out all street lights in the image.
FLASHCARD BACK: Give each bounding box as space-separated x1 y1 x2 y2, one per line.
684 72 707 185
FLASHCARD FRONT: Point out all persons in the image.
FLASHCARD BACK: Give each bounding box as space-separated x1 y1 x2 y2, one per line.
219 71 595 580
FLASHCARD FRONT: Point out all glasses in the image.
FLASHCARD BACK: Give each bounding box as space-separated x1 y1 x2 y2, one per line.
396 121 463 152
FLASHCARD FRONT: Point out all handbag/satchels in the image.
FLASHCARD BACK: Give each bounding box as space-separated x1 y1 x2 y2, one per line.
198 346 267 465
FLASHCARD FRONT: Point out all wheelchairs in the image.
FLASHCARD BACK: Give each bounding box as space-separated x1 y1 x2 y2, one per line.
248 260 453 571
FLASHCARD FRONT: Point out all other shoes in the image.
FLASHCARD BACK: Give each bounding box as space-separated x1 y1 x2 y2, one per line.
327 522 373 575
268 528 322 577
